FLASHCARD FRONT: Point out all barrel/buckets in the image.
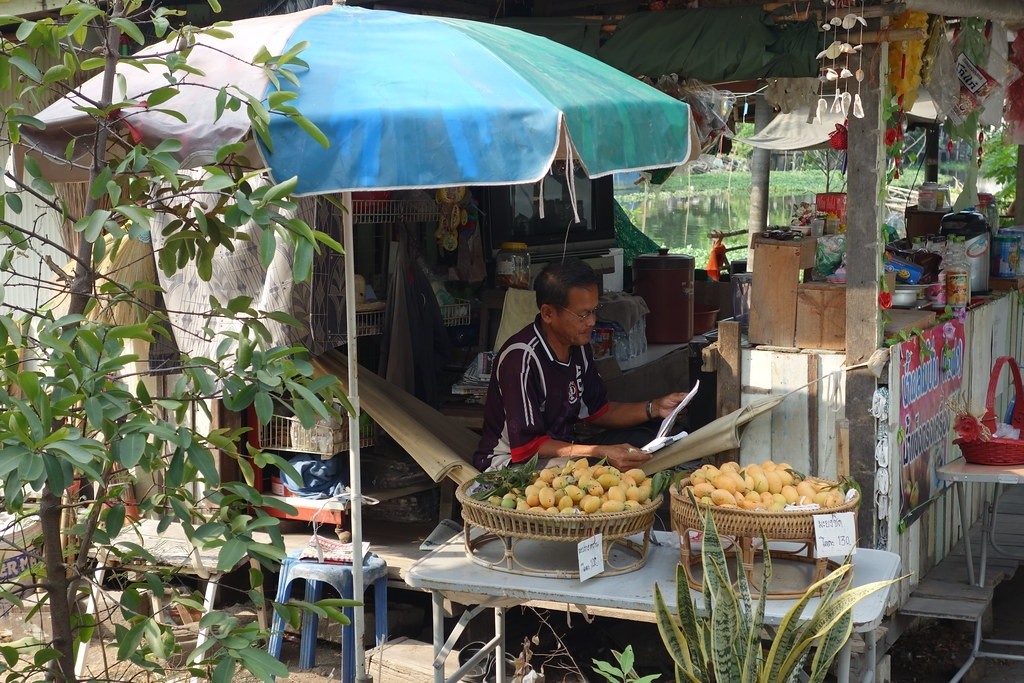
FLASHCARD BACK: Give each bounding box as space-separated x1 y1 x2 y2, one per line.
939 211 990 295
0 511 41 595
991 225 1024 278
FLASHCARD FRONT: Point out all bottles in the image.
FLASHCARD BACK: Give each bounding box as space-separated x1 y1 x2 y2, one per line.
495 242 531 291
594 312 647 361
910 233 972 308
975 192 999 236
918 181 951 212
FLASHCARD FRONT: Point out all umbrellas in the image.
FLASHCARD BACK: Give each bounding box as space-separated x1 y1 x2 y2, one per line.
17 1 694 683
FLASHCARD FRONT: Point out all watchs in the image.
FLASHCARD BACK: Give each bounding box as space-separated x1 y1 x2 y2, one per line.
646 398 654 418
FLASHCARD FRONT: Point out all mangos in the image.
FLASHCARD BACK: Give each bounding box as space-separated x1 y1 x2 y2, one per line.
487 459 654 514
680 461 845 511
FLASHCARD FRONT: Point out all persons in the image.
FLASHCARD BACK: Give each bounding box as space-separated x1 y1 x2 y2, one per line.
475 258 689 471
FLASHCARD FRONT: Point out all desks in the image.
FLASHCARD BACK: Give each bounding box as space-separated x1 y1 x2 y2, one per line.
936 454 1024 587
66 518 267 683
405 519 901 683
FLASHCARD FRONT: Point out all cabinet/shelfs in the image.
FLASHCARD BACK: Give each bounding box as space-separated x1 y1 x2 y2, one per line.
259 191 471 456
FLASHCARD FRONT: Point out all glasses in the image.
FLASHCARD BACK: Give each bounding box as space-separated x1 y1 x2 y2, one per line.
556 302 605 320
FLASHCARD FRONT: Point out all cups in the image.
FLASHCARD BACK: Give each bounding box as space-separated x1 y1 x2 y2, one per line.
811 220 825 237
828 218 840 234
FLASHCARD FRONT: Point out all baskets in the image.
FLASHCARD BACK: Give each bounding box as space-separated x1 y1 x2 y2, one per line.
455 470 664 579
952 356 1024 466
669 476 860 600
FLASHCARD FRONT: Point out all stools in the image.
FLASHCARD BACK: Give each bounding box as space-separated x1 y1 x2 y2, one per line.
268 553 388 683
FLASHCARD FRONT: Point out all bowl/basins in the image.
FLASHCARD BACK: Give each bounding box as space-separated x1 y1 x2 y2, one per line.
694 304 720 336
891 290 920 309
